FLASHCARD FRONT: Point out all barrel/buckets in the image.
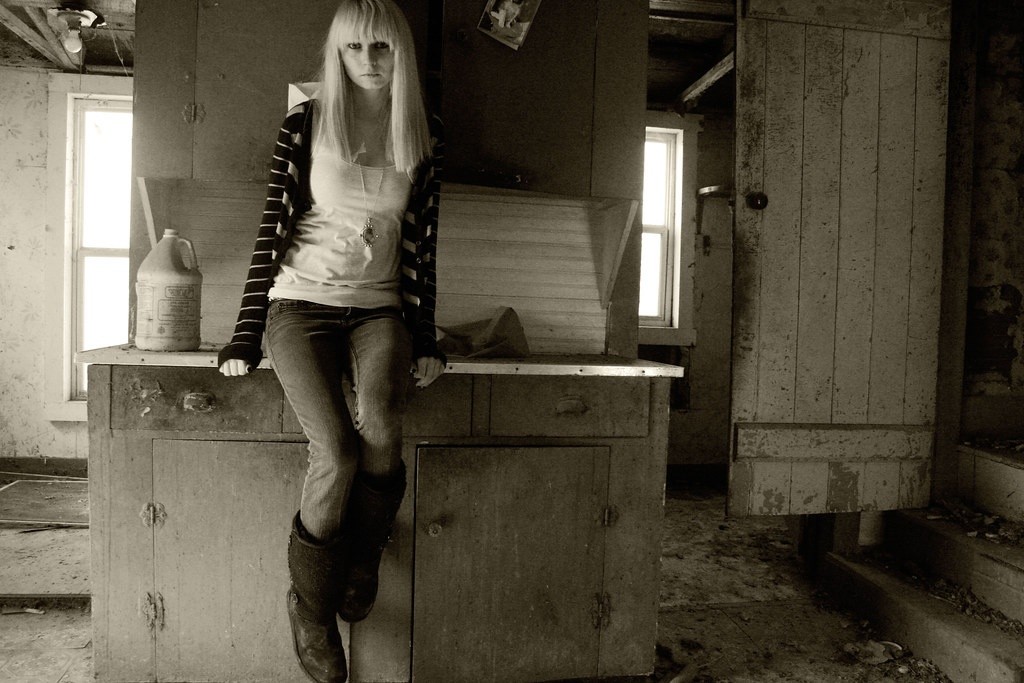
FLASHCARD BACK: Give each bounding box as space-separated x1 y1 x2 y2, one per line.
135 229 203 351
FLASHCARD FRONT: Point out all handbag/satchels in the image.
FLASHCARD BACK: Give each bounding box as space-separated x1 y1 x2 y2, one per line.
435 306 530 358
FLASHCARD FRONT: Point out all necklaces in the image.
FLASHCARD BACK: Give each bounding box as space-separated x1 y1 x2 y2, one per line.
356 158 386 248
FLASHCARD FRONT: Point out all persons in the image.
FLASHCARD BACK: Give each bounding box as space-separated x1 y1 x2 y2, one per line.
217 1 447 683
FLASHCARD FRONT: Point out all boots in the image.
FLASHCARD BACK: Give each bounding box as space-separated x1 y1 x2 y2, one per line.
286 510 348 683
338 459 407 624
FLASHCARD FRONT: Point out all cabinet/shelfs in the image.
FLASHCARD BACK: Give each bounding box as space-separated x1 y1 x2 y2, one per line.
76 340 684 683
